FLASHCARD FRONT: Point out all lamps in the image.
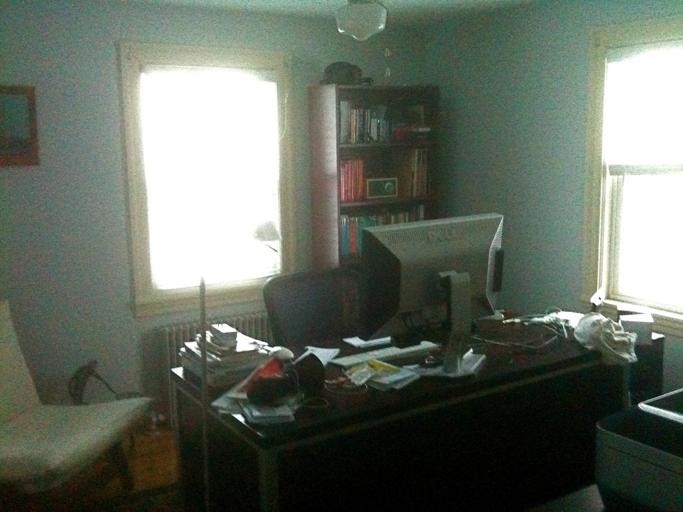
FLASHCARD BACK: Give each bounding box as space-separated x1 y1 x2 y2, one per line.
335 0 390 42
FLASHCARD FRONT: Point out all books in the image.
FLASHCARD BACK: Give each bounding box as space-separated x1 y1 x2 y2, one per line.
177 326 272 391
339 99 431 258
347 360 416 393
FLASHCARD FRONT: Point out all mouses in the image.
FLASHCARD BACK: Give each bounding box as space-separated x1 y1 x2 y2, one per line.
269 346 294 360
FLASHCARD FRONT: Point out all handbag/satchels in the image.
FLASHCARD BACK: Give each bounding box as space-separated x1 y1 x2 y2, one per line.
285 353 330 393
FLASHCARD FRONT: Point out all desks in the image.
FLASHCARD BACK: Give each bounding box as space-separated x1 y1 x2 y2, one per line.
166 310 628 511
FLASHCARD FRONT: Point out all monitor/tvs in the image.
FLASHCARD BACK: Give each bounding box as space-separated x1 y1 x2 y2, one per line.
357 212 504 380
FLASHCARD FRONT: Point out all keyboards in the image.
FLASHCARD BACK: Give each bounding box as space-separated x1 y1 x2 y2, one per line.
328 340 439 368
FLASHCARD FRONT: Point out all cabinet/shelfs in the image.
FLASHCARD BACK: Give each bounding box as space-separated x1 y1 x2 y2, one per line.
309 79 442 270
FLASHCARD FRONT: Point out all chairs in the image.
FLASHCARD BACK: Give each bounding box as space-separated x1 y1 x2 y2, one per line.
263 267 363 346
0 297 157 509
